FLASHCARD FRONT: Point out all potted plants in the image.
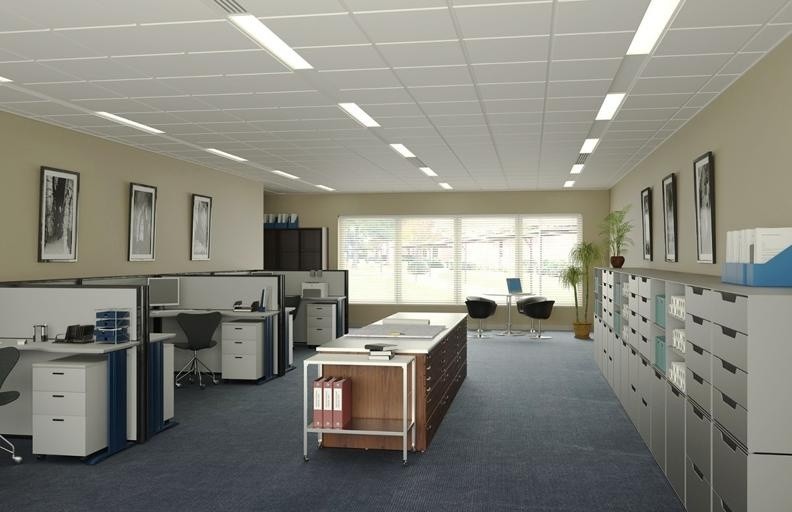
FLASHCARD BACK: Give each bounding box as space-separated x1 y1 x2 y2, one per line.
640 186 657 263
128 182 158 263
660 173 679 263
692 151 717 265
39 165 80 263
190 194 212 261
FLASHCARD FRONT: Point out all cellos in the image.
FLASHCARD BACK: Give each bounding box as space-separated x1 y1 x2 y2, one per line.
301 282 329 298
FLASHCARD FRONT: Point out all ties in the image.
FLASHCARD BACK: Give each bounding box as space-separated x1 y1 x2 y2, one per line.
148 278 180 309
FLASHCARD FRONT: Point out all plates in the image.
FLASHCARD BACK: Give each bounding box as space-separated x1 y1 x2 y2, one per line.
30 356 110 464
315 311 477 454
305 304 337 346
219 318 264 382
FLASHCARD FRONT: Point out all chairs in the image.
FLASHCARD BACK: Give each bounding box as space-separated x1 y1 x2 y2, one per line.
0 346 24 465
169 312 224 389
464 294 556 340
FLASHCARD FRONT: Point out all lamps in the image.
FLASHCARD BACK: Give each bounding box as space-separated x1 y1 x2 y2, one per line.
169 312 224 389
0 346 24 465
464 294 556 340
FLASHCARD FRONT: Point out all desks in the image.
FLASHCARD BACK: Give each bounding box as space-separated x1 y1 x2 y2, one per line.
0 336 131 355
147 306 274 322
303 353 417 467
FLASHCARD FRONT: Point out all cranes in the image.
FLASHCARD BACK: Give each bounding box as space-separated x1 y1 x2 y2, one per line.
312 376 352 428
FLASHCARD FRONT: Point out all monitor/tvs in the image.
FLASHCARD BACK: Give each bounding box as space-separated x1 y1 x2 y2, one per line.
365 343 399 361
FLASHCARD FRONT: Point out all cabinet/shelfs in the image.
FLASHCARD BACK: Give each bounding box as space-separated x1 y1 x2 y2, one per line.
613 272 712 451
305 304 337 346
651 277 792 512
315 311 477 454
30 356 110 464
594 268 613 388
219 318 264 382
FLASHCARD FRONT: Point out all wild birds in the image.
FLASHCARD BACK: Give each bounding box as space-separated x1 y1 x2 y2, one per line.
506 278 533 296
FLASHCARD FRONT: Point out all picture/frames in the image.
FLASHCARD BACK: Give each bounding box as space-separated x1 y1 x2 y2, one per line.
640 186 657 263
39 165 80 263
660 173 679 263
0 336 131 355
190 194 212 261
147 306 274 322
692 151 717 265
303 353 417 467
128 182 158 263
